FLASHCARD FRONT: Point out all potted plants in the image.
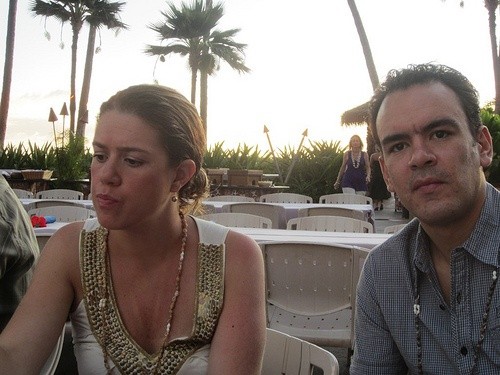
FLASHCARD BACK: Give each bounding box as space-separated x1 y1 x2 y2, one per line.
202 141 229 186
222 142 275 185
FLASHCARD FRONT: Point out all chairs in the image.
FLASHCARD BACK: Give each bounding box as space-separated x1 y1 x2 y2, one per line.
11 189 408 375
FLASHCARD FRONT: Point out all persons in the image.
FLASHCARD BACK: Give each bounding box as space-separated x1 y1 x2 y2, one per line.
0 84 267 374
350 60 500 374
0 164 41 334
333 135 371 196
369 141 392 212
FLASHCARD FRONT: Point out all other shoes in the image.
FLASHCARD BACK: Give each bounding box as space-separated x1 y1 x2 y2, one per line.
374 203 384 210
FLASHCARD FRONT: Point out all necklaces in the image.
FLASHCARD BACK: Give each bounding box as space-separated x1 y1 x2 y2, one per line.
351 150 361 168
412 261 499 374
99 206 189 374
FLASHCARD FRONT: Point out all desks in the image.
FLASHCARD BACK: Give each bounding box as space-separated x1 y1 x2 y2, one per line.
19 198 376 234
32 222 393 251
5 179 89 200
